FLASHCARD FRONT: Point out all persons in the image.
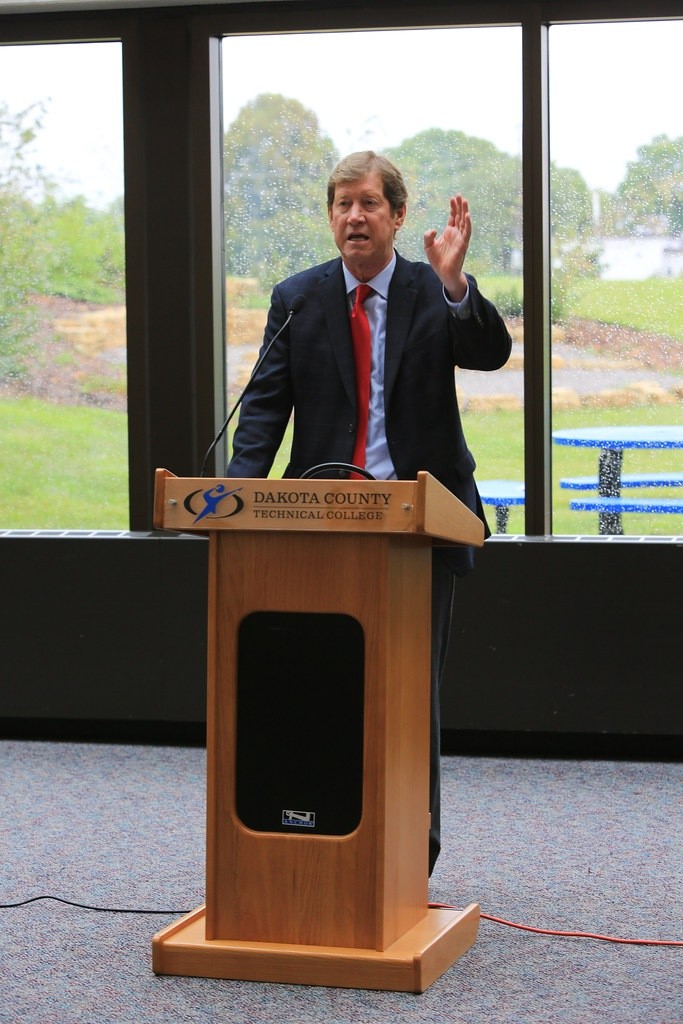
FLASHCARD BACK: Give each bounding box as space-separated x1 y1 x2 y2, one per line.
224 150 512 877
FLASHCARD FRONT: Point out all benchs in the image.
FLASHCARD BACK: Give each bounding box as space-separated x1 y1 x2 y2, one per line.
560 472 683 513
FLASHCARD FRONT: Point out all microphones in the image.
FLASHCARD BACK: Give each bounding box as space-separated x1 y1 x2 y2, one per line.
200 294 306 478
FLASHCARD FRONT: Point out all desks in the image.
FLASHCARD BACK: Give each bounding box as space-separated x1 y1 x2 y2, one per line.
552 426 683 535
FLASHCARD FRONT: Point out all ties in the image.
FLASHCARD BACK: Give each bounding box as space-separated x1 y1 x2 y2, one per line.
350 284 374 480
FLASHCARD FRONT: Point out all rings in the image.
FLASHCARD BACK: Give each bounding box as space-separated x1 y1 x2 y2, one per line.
458 226 464 231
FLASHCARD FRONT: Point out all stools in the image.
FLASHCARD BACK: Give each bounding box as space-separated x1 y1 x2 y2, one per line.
476 480 525 534
